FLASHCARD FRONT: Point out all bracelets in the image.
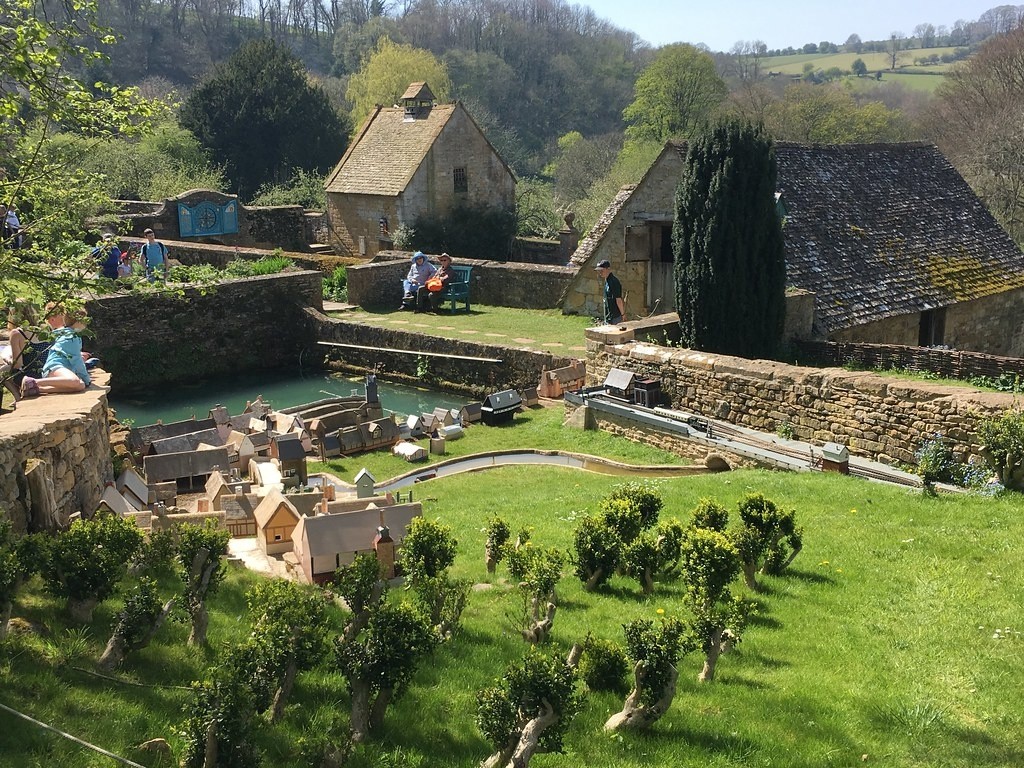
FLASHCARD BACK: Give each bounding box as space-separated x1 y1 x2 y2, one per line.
146 266 150 269
621 313 626 316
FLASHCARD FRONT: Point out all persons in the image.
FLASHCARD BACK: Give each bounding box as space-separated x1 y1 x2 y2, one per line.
0 203 20 249
594 260 628 326
414 253 457 315
93 232 132 280
138 228 170 283
0 297 100 407
397 251 437 311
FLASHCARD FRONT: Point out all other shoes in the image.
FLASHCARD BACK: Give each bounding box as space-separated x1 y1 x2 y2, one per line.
402 294 414 301
398 305 409 311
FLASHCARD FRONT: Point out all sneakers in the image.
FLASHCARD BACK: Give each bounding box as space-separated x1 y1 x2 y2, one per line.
22 375 37 391
20 387 39 396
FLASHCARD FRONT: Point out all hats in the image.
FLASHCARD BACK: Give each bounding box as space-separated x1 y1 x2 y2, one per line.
412 252 427 263
438 253 453 262
594 260 611 271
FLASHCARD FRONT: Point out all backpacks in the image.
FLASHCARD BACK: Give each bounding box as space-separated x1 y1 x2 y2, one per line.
428 279 442 292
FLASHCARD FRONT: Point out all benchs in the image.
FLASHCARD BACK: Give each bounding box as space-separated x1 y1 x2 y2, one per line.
400 264 474 315
